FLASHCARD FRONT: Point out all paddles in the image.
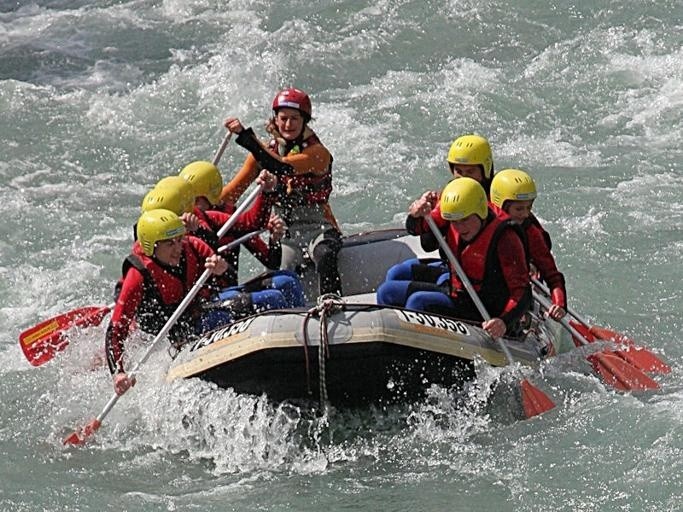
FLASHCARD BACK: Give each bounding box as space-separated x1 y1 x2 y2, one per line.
424 214 556 417
530 274 673 389
63 269 212 446
19 219 270 367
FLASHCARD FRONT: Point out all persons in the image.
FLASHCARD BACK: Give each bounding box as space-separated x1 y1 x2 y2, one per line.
103 206 310 395
386 167 569 324
155 168 279 289
376 175 531 339
401 133 495 267
218 86 344 296
176 161 286 289
87 184 301 376
420 210 424 214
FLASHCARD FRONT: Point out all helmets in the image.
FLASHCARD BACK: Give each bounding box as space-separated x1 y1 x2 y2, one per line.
273 88 310 121
439 177 488 221
489 168 537 209
136 160 223 257
439 135 492 179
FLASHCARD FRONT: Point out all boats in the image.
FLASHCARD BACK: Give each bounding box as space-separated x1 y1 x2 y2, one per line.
164 228 555 421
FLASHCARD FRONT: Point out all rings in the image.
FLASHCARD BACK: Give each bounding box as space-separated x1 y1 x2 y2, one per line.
123 381 129 387
